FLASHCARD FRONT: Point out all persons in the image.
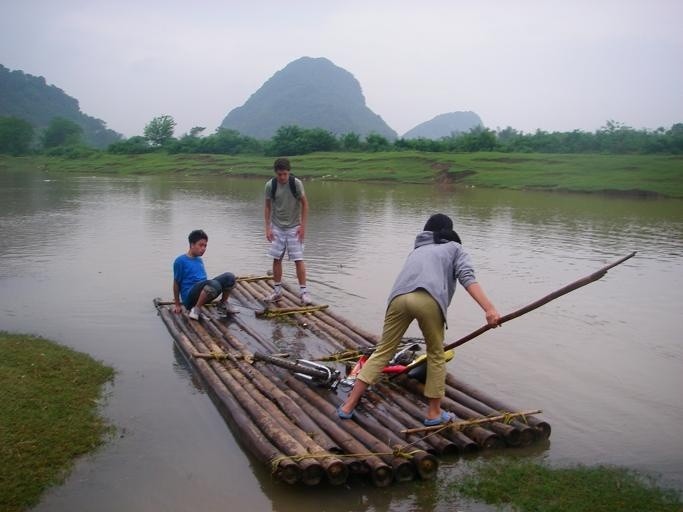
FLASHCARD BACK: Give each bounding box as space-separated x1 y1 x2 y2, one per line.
328 208 502 425
167 228 241 321
261 157 312 307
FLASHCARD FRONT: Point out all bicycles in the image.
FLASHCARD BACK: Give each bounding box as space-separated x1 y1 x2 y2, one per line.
254 336 453 394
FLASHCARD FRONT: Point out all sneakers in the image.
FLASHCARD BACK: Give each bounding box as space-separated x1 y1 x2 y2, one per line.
217 300 240 313
264 290 282 302
189 307 199 320
299 291 312 304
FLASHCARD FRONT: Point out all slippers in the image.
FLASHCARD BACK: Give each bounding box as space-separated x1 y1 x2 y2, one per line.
339 402 354 419
424 411 455 425
